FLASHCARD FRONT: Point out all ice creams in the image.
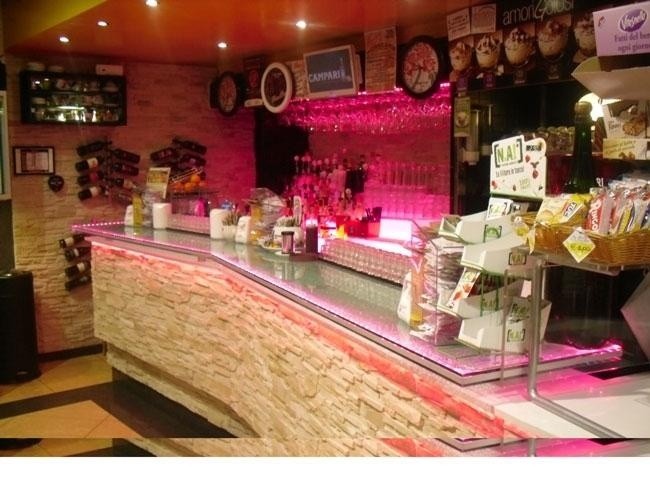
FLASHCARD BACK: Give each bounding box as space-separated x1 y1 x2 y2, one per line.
572 11 597 57
475 35 502 74
449 42 473 75
504 28 535 68
536 20 571 59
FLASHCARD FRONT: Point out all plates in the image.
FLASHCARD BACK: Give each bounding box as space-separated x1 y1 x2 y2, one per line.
48 65 65 71
27 61 45 71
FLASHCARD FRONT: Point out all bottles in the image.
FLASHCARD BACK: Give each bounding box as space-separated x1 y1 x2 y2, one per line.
74 156 110 172
176 154 206 168
63 246 92 260
106 147 139 165
104 189 133 207
58 232 85 249
106 161 138 177
172 139 207 155
101 175 136 188
289 148 369 223
150 147 182 162
64 261 91 278
281 229 295 254
77 184 115 202
63 277 91 291
306 218 318 253
75 139 112 157
77 170 108 186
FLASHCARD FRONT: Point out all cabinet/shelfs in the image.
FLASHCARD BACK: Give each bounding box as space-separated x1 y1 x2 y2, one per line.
0 270 41 386
19 68 128 125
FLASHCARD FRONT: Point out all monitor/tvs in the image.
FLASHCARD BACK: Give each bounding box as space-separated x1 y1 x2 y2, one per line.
302 44 363 98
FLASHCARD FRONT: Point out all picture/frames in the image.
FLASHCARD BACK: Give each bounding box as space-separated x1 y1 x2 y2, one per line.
260 61 293 113
213 70 245 115
396 35 442 98
12 145 55 174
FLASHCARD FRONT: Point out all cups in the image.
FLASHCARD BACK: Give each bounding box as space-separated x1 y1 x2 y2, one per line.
209 207 233 239
151 201 174 230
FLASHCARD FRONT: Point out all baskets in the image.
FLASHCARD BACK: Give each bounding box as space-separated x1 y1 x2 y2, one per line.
515 212 649 266
